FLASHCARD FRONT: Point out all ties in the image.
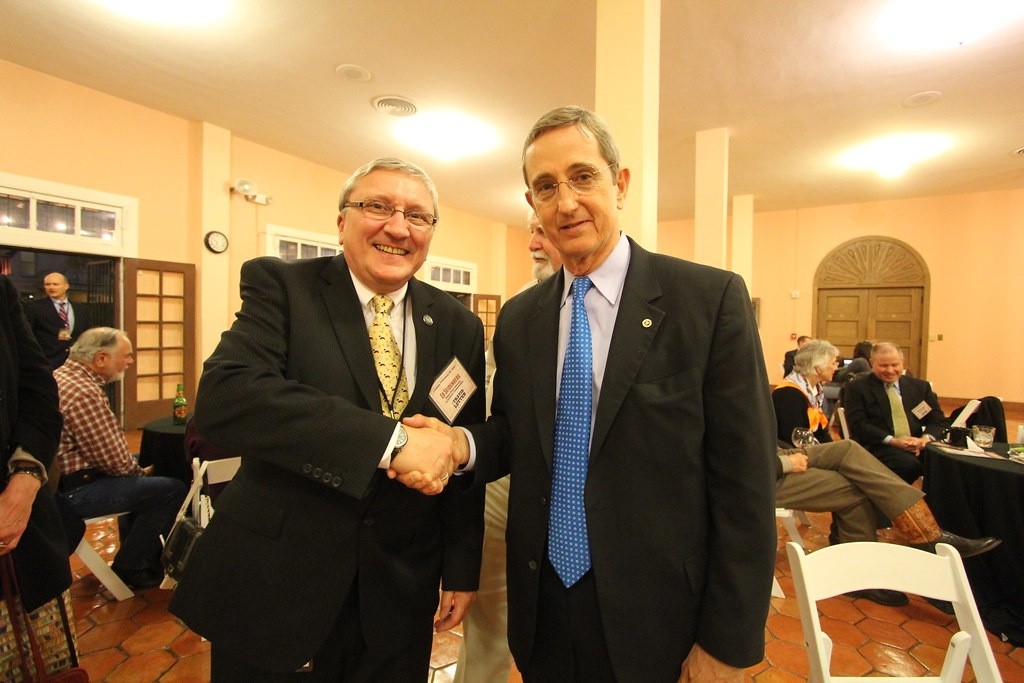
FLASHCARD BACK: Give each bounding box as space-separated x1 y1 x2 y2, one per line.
547 276 597 590
58 301 69 331
887 382 911 437
369 293 411 420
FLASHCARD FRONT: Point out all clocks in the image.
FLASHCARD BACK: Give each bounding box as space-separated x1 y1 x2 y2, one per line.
204 230 229 253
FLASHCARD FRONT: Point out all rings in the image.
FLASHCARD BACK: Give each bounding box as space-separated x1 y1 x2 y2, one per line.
441 472 448 481
0 542 6 547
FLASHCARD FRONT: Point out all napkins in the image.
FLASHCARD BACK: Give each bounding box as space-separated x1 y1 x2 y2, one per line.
966 436 985 454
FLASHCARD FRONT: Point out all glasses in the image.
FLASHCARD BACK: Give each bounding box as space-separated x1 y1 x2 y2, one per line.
530 162 614 203
342 197 437 230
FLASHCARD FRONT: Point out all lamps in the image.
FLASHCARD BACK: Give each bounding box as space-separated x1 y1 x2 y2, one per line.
230 178 273 205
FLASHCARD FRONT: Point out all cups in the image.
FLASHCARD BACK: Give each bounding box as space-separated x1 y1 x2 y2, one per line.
972 425 996 449
792 427 814 447
942 427 972 446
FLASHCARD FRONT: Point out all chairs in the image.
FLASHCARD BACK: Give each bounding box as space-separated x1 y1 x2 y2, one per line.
771 407 1004 683
46 455 243 601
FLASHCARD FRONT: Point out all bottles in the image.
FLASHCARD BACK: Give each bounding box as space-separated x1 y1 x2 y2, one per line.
173 384 187 425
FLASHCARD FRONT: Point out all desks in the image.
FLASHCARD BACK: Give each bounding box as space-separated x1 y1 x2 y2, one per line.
135 411 194 474
922 440 1024 648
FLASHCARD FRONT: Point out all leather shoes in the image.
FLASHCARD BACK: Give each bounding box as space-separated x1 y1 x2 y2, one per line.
911 530 1002 557
857 587 910 605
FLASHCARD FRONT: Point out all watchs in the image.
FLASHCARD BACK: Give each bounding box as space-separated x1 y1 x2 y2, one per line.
390 426 408 461
9 467 44 482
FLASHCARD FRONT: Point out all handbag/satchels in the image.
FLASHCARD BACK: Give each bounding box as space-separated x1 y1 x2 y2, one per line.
161 516 205 577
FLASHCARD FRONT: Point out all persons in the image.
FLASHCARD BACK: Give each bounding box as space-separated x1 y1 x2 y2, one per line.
772 339 839 542
776 439 1002 607
388 106 779 683
832 340 873 381
836 341 948 528
52 326 188 589
166 157 485 683
783 336 812 379
0 271 87 683
24 273 86 366
451 211 563 683
